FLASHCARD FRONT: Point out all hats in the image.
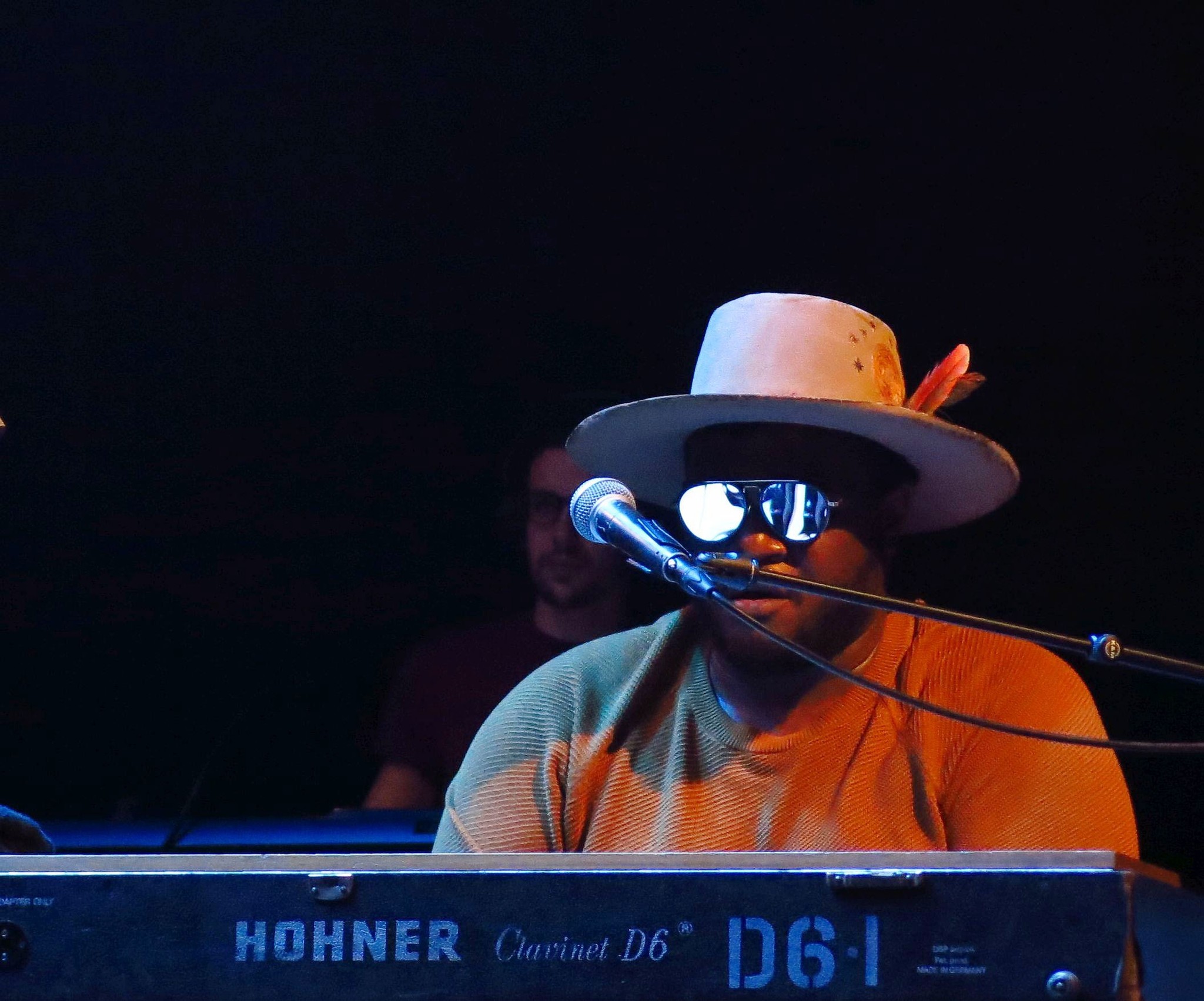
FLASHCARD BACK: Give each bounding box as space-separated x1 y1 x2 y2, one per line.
563 293 1019 538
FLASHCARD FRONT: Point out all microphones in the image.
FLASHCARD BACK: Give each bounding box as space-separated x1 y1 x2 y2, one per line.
569 475 722 605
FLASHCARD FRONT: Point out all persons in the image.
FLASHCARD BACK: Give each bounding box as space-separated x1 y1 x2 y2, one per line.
432 292 1139 860
364 416 628 807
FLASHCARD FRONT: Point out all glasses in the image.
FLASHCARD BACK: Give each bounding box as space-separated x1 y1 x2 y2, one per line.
674 478 864 542
